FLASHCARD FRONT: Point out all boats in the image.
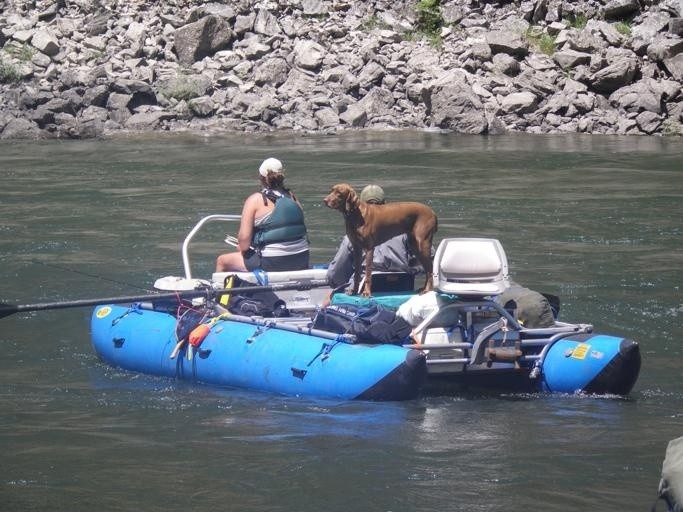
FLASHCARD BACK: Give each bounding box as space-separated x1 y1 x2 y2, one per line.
89 214 640 401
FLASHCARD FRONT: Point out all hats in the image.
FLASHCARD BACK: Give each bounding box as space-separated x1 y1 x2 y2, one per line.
259 158 283 178
360 186 384 204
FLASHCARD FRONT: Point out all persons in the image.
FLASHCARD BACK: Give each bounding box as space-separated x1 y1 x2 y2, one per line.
216 157 310 273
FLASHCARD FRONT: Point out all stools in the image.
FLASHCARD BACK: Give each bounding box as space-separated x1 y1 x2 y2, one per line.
211 269 333 316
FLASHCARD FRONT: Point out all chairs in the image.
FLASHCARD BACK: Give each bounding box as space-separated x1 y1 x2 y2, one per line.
403 236 522 375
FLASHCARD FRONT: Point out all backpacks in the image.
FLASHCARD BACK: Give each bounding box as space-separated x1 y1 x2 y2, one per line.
308 303 412 344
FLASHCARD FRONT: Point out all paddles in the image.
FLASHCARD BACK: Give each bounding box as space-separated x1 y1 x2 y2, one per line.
0 276 332 317
